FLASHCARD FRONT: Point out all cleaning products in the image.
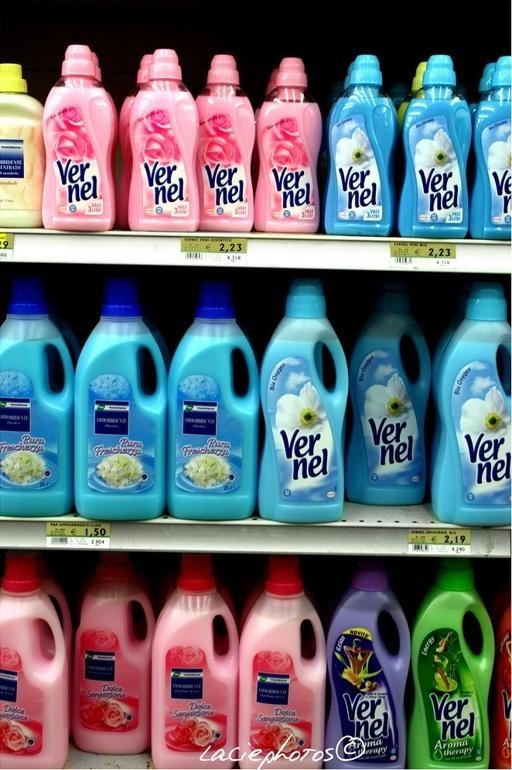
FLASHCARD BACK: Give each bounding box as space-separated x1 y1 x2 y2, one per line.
92 52 106 85
117 54 155 228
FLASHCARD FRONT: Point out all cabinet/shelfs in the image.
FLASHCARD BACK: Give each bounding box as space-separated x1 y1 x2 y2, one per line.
0 225 511 770
